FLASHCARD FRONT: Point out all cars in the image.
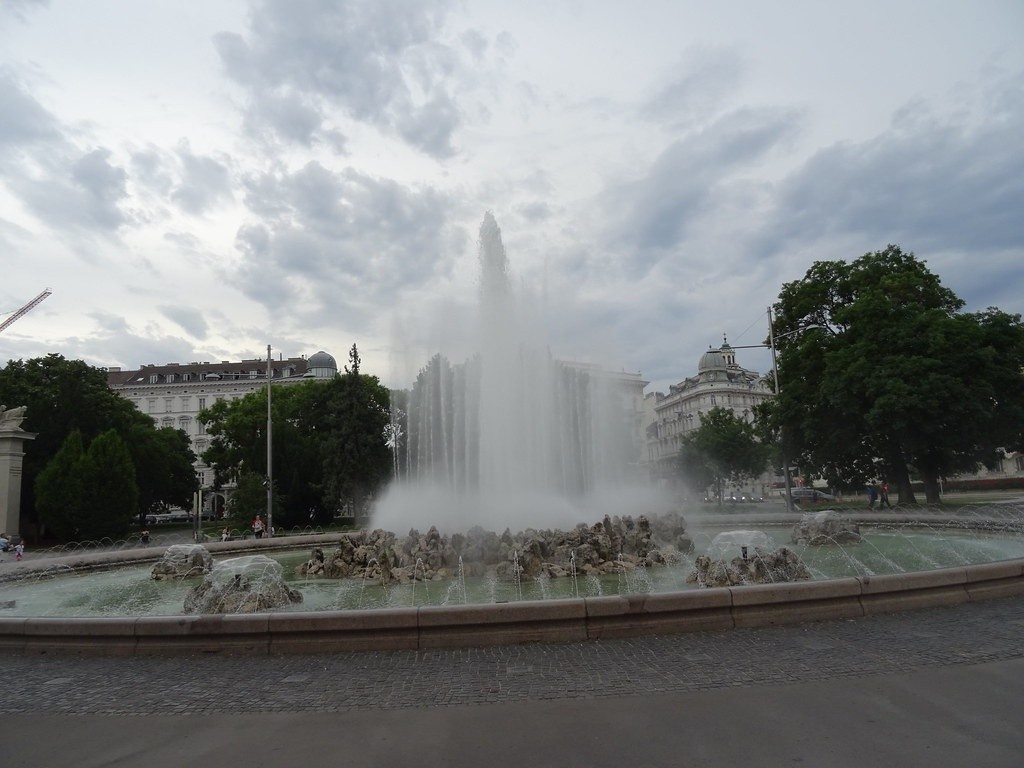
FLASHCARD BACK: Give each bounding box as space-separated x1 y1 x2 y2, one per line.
131 512 219 526
791 488 838 505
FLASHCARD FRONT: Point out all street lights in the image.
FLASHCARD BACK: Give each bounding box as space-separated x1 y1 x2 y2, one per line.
205 344 316 537
706 304 820 512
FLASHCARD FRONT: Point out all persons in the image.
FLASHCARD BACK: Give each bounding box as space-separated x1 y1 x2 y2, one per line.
141 528 149 548
222 526 230 542
252 515 264 539
0 533 24 563
868 480 892 510
